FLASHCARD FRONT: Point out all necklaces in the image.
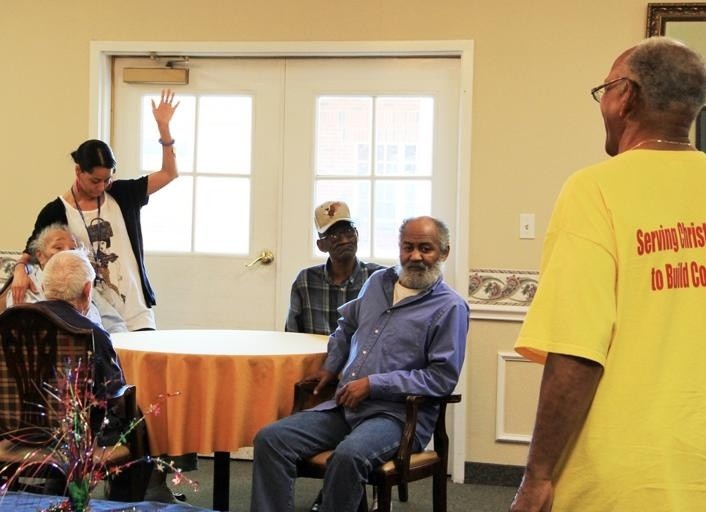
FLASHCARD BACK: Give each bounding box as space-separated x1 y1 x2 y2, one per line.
71 186 102 286
631 139 691 149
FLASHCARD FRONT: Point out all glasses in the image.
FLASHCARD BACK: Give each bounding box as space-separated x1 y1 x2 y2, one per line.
320 223 354 241
591 77 628 102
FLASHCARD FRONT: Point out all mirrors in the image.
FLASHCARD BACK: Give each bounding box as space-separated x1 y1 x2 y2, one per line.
644 2 706 154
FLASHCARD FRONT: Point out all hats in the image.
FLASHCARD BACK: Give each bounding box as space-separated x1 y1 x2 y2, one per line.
314 200 354 234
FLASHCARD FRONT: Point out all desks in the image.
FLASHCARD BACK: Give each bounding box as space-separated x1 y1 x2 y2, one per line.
105 328 332 512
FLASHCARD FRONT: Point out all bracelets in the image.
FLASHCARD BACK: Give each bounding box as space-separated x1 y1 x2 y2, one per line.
159 139 175 147
14 262 30 276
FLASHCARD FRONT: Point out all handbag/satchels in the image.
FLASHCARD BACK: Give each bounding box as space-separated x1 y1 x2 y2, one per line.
88 219 112 242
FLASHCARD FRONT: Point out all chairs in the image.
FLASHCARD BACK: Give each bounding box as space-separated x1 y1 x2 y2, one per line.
0 304 139 502
289 379 462 512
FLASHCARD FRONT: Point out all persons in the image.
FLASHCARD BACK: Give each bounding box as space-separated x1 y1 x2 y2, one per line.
249 215 469 512
0 242 186 501
11 87 181 332
509 35 705 512
5 222 130 334
284 201 391 512
